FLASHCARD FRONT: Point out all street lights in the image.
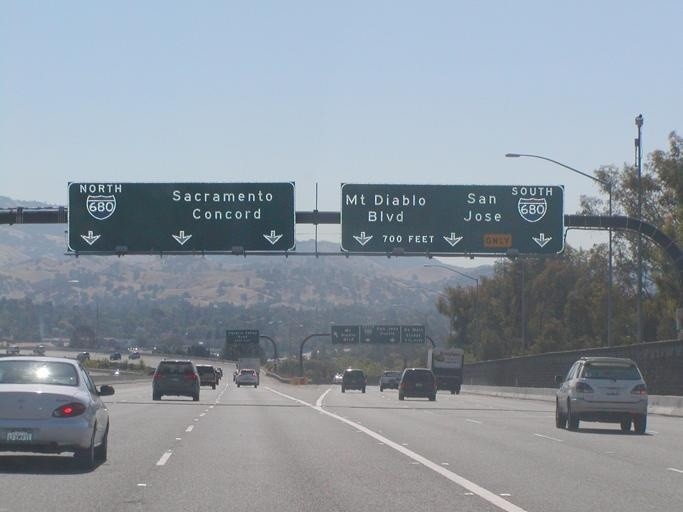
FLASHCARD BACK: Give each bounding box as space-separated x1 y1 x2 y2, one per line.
633 113 645 341
407 286 455 338
422 263 479 363
504 152 613 349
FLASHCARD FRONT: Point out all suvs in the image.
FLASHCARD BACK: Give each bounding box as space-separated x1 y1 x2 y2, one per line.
377 370 402 392
549 355 652 435
340 368 367 393
148 358 202 402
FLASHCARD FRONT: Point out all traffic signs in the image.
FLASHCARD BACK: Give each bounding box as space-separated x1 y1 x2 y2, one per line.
331 324 427 345
225 328 259 345
67 179 297 255
340 181 567 258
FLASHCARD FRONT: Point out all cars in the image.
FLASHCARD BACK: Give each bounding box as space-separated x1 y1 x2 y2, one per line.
235 369 258 388
332 372 344 384
4 342 141 363
397 367 439 402
0 355 117 470
232 369 237 380
195 364 223 390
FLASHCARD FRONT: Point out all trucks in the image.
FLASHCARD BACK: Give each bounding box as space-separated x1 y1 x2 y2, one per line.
235 357 261 385
425 347 465 395
